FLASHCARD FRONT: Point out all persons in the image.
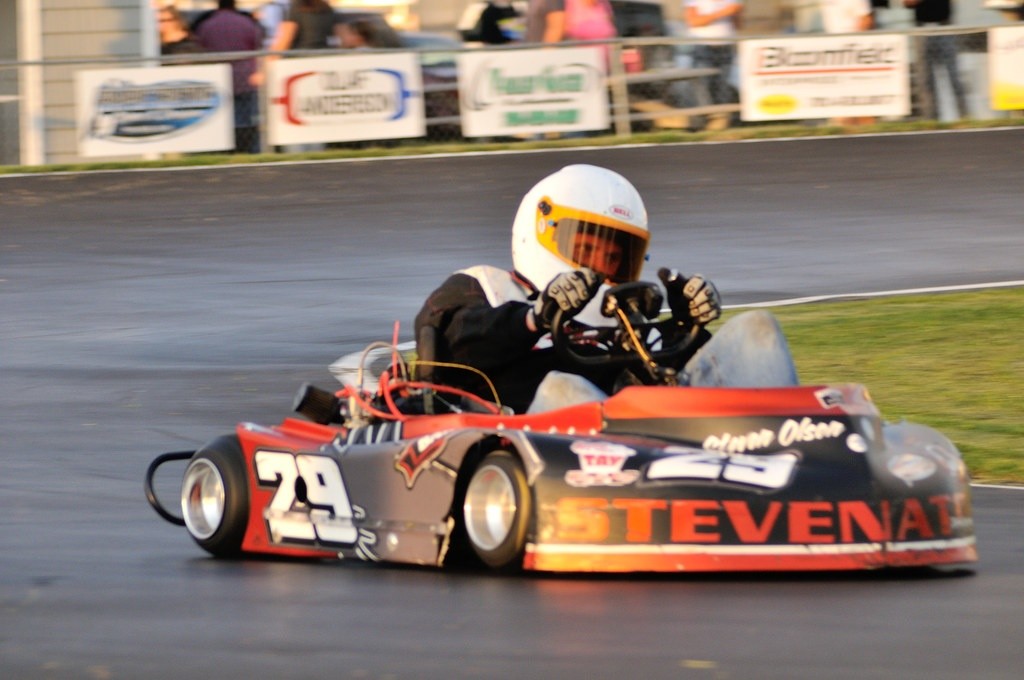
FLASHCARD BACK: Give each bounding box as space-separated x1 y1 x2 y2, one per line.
356 163 801 417
157 1 1023 153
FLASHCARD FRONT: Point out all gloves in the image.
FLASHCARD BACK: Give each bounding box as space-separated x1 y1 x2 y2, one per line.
658 268 721 327
533 266 601 335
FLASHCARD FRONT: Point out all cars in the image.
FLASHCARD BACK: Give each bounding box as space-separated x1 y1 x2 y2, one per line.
228 5 477 128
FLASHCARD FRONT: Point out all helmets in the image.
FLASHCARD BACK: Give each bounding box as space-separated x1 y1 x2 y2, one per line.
512 163 649 298
